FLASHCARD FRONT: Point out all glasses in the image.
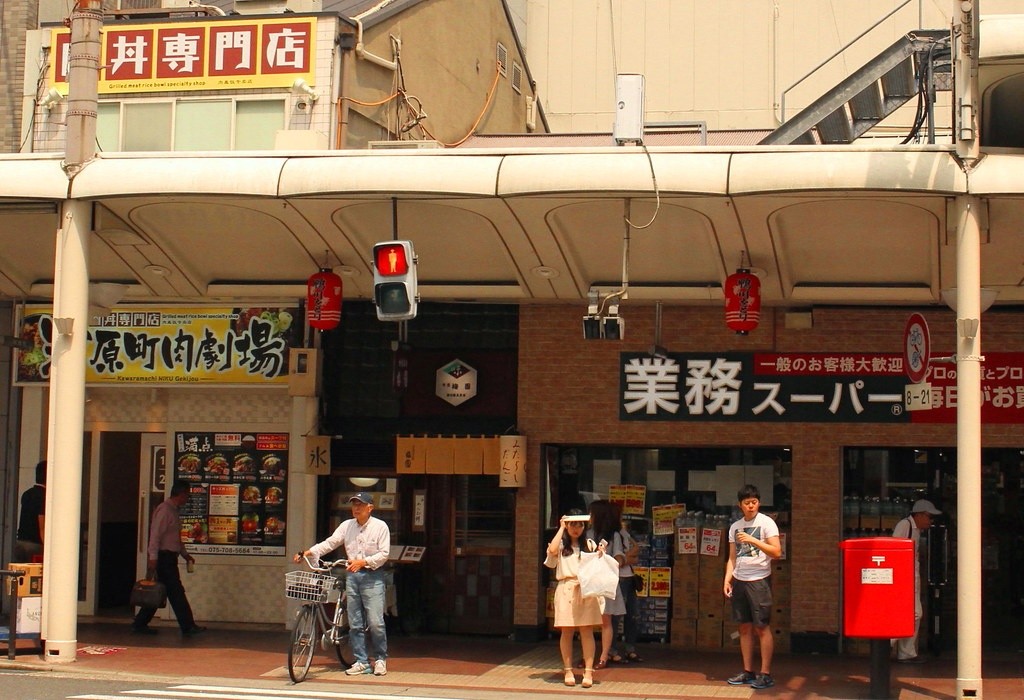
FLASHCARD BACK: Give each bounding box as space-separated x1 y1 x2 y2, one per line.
923 513 934 519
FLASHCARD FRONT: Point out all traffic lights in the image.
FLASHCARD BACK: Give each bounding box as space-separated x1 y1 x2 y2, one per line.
371 241 421 323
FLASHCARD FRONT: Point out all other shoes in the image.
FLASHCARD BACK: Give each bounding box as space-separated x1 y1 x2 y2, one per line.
899 656 927 664
184 626 206 636
133 626 158 634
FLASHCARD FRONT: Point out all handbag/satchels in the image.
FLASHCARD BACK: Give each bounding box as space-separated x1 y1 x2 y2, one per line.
130 570 167 608
634 574 645 591
578 549 620 600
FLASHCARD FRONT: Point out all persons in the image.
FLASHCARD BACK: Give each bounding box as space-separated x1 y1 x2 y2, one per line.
894 500 943 666
543 509 599 689
723 484 782 691
128 478 209 636
16 457 49 563
294 493 392 676
588 499 643 669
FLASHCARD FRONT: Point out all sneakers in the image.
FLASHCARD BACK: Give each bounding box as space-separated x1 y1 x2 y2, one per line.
345 662 373 675
727 671 756 685
752 673 775 688
374 660 387 675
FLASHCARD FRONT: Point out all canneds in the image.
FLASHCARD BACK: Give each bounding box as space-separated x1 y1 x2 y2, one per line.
187 559 193 573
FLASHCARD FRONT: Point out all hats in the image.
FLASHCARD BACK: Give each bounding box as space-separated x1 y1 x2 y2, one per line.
912 499 943 516
349 492 373 504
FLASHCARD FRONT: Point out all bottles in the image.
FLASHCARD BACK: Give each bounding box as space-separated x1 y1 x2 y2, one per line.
843 494 909 537
674 509 727 529
187 560 194 573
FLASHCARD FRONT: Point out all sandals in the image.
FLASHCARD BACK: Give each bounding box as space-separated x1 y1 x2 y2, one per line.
625 652 644 662
608 653 628 664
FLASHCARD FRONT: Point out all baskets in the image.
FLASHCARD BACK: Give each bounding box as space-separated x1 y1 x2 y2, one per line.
284 570 337 603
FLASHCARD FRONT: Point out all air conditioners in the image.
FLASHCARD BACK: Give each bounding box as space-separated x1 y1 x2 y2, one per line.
368 140 444 149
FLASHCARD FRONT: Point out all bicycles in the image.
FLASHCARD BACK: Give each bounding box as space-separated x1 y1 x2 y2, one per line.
286 549 372 684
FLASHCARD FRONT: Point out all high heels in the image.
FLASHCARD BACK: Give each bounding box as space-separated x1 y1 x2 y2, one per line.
564 667 576 686
582 669 595 687
594 659 609 669
579 660 585 667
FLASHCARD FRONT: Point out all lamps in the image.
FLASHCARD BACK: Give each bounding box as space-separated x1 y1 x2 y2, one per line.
601 291 625 340
39 87 64 110
581 288 603 340
293 78 320 100
